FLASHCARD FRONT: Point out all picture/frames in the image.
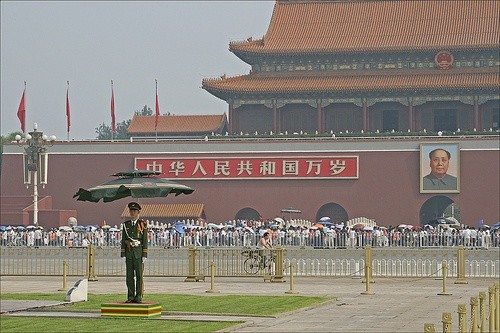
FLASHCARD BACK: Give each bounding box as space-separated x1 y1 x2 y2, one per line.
419 142 461 194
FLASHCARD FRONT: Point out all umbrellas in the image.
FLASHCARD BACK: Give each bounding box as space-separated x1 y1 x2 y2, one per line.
398 224 406 228
102 225 111 229
207 223 217 227
245 226 254 233
315 224 323 229
274 218 284 223
26 226 36 228
73 168 194 215
59 226 72 231
175 226 184 233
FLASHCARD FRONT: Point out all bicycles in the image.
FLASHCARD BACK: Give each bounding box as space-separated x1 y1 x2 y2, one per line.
243 253 287 274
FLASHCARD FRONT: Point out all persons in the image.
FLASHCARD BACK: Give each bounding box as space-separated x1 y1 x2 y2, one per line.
0 223 121 249
120 202 148 302
147 215 500 249
257 232 272 268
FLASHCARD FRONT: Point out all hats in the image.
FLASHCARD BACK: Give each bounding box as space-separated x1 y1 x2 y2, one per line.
128 202 142 211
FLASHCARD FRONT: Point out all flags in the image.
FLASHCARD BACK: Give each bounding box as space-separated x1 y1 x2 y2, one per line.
111 92 116 132
17 94 26 133
155 95 160 126
66 95 71 131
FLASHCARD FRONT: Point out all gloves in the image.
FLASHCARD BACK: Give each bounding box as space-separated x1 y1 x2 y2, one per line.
121 256 126 262
142 257 147 263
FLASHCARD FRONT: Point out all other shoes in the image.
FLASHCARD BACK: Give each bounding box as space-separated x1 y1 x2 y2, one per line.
125 299 135 303
136 298 142 302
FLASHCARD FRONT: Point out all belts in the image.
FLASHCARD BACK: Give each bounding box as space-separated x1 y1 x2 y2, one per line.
130 241 141 245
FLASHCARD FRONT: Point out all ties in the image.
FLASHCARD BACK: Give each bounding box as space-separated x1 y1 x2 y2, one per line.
132 221 135 229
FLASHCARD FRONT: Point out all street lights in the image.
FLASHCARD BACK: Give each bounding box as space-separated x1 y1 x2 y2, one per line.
13 122 56 226
